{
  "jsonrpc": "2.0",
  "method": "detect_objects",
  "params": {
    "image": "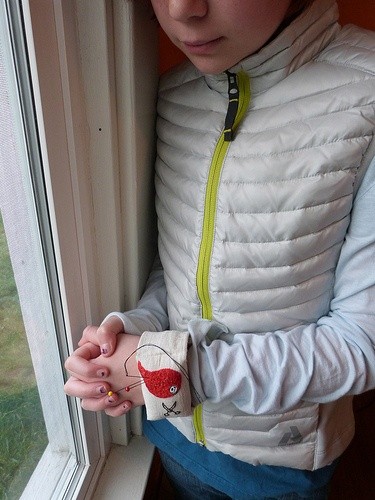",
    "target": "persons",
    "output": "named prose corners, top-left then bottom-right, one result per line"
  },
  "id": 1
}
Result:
top-left (62, 1), bottom-right (375, 500)
